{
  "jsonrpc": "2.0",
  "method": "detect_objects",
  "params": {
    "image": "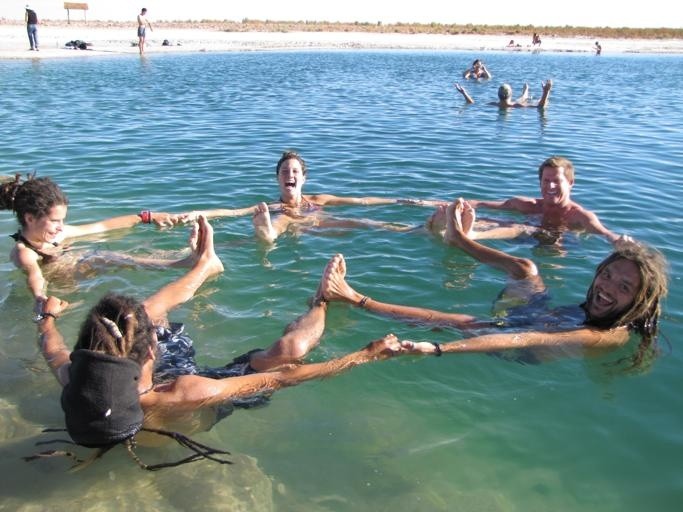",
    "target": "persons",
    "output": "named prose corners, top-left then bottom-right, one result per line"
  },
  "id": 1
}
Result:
top-left (463, 59), bottom-right (491, 80)
top-left (508, 40), bottom-right (515, 47)
top-left (532, 32), bottom-right (541, 47)
top-left (593, 41), bottom-right (601, 56)
top-left (454, 78), bottom-right (553, 109)
top-left (25, 4), bottom-right (39, 51)
top-left (138, 7), bottom-right (153, 56)
